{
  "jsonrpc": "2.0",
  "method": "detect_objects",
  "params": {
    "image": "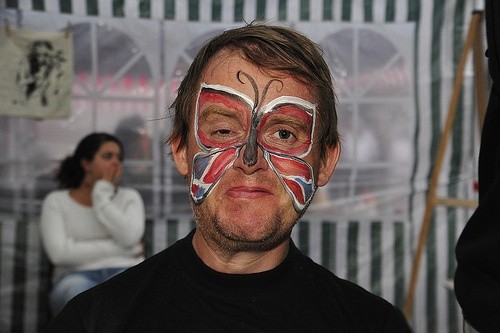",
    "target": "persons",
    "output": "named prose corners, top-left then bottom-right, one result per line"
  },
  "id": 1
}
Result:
top-left (46, 17), bottom-right (419, 331)
top-left (37, 130), bottom-right (145, 318)
